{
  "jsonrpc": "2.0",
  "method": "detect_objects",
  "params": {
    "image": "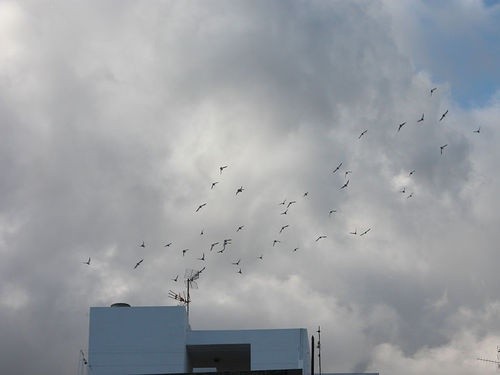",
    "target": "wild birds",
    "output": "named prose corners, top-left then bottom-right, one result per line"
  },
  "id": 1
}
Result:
top-left (417, 113), bottom-right (424, 123)
top-left (438, 110), bottom-right (449, 121)
top-left (344, 171), bottom-right (352, 179)
top-left (133, 179), bottom-right (372, 282)
top-left (332, 162), bottom-right (342, 174)
top-left (472, 126), bottom-right (481, 134)
top-left (430, 88), bottom-right (437, 96)
top-left (358, 130), bottom-right (368, 140)
top-left (439, 144), bottom-right (447, 155)
top-left (219, 166), bottom-right (227, 174)
top-left (397, 122), bottom-right (407, 132)
top-left (399, 170), bottom-right (417, 199)
top-left (83, 257), bottom-right (91, 265)
